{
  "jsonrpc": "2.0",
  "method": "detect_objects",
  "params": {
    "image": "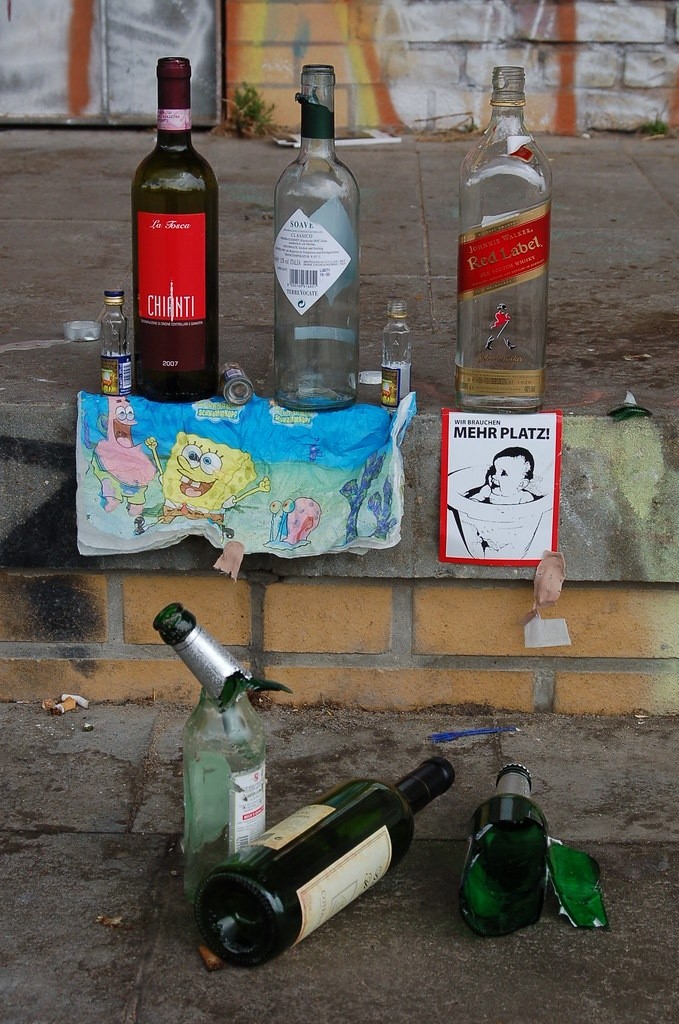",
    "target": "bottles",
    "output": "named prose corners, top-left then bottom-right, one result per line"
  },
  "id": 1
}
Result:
top-left (273, 65), bottom-right (358, 412)
top-left (380, 300), bottom-right (412, 412)
top-left (132, 57), bottom-right (219, 405)
top-left (153, 602), bottom-right (268, 903)
top-left (193, 757), bottom-right (455, 969)
top-left (455, 68), bottom-right (552, 414)
top-left (458, 762), bottom-right (550, 939)
top-left (101, 289), bottom-right (133, 396)
top-left (220, 361), bottom-right (253, 405)
